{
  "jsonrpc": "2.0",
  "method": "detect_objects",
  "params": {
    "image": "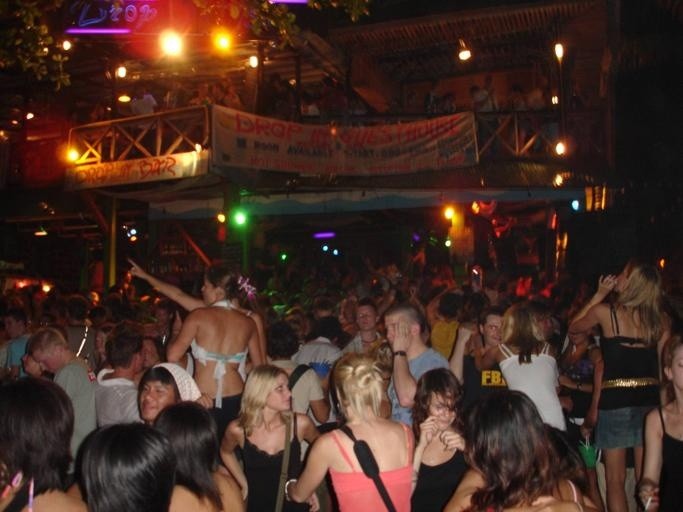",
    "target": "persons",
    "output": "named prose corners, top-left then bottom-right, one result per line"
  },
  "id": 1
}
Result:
top-left (0, 66), bottom-right (683, 511)
